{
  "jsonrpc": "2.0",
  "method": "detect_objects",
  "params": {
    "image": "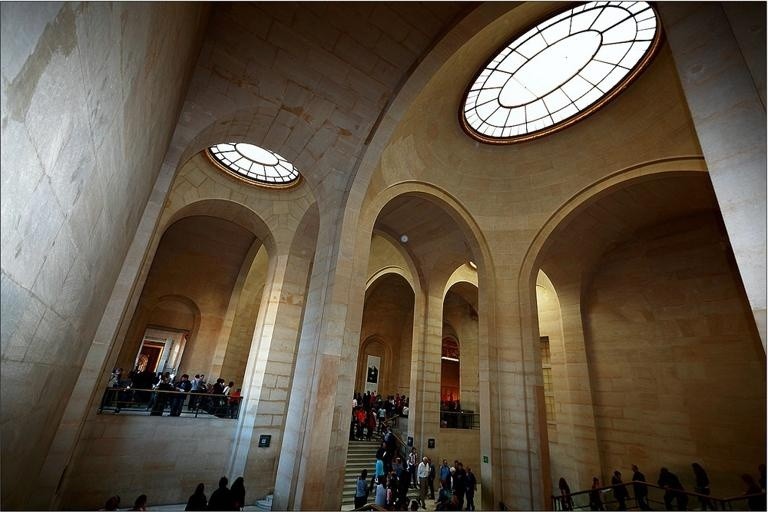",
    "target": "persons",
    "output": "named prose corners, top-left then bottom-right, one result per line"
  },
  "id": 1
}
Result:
top-left (128, 494), bottom-right (147, 511)
top-left (558, 462), bottom-right (766, 511)
top-left (349, 391), bottom-right (477, 512)
top-left (185, 476), bottom-right (245, 511)
top-left (101, 368), bottom-right (241, 419)
top-left (99, 495), bottom-right (120, 511)
